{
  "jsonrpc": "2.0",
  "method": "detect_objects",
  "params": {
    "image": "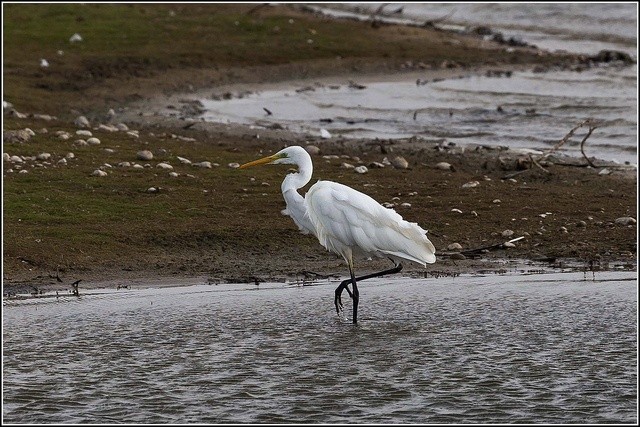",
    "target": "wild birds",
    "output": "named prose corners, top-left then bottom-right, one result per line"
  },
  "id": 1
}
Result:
top-left (241, 145), bottom-right (526, 332)
